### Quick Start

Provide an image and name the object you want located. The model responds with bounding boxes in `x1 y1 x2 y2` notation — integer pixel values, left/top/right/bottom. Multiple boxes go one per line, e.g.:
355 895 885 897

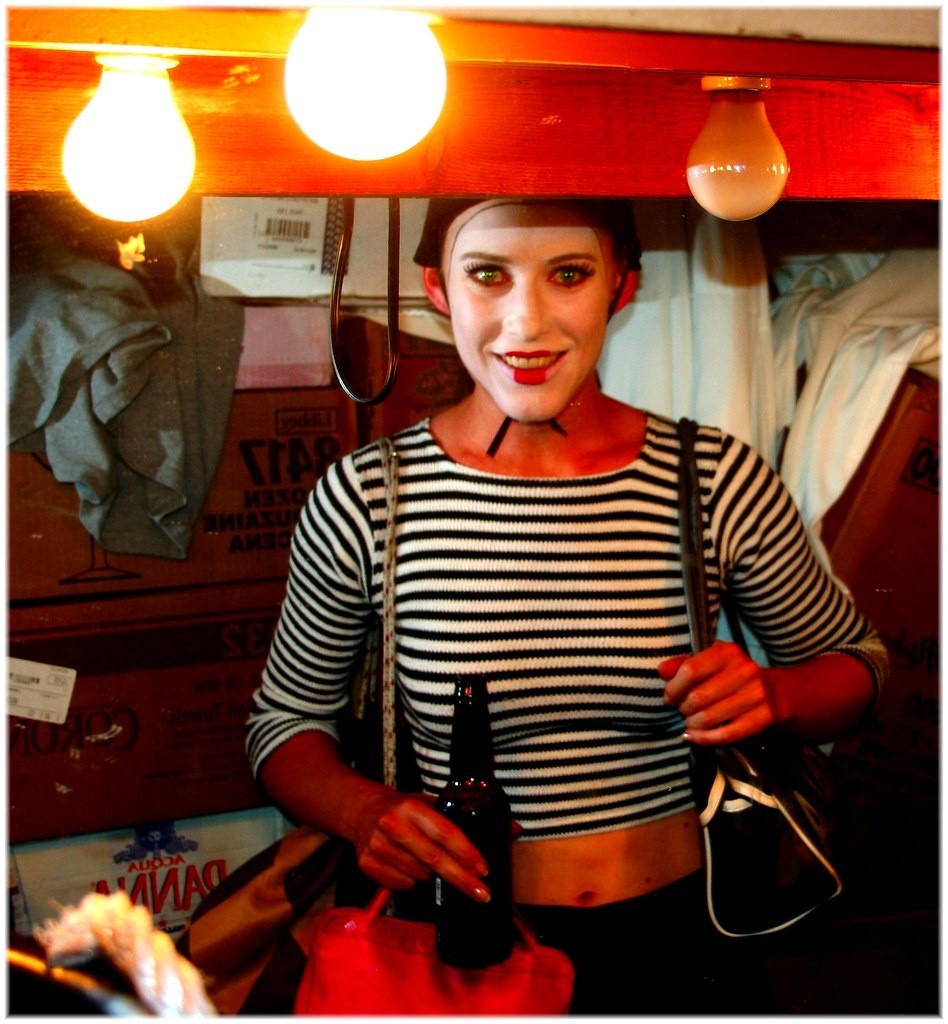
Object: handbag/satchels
678 415 846 938
177 824 393 1014
296 887 574 1017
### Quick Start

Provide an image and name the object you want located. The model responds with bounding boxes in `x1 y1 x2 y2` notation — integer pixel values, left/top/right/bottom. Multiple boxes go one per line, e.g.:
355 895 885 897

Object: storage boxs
8 191 477 969
779 362 940 810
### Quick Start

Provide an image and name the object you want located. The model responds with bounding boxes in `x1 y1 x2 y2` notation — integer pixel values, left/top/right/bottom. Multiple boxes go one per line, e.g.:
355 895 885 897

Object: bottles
435 678 516 971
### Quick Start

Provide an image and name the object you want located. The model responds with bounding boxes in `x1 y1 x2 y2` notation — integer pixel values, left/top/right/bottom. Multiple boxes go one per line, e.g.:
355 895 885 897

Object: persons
244 198 889 1017
